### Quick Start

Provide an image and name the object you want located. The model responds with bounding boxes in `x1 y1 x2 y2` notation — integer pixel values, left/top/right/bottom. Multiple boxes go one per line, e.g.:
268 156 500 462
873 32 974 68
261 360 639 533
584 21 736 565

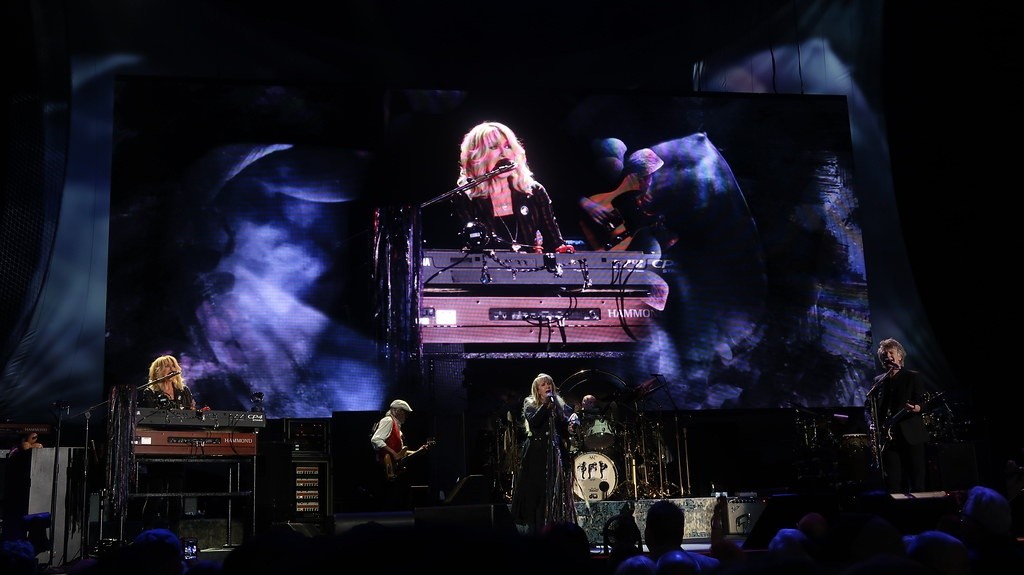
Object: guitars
867 399 916 453
384 439 437 480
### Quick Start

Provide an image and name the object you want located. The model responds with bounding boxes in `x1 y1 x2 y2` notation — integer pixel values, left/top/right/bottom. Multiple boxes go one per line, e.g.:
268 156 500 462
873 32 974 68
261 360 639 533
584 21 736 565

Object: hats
390 399 413 413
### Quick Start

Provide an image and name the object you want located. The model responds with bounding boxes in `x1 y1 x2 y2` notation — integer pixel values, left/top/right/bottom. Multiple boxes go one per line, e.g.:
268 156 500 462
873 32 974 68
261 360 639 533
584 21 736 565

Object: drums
579 415 616 449
571 452 620 502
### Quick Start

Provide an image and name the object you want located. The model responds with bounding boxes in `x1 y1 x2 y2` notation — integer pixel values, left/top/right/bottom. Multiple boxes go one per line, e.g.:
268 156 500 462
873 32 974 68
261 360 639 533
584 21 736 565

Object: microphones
169 371 181 375
547 393 554 403
885 358 899 368
495 157 518 171
651 373 663 376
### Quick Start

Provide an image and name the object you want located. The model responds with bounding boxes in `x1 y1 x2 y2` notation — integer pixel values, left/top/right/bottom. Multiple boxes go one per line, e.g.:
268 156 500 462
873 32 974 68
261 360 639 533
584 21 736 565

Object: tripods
616 396 665 501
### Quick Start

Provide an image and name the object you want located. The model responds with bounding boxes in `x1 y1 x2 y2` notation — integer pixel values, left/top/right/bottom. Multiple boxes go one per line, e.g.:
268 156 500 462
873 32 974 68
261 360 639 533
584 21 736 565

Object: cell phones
182 537 198 565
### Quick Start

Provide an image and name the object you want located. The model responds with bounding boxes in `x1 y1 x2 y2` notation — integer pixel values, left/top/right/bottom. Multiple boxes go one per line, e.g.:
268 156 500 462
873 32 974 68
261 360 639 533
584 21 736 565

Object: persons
370 399 433 512
568 394 598 426
415 120 568 255
865 338 933 474
141 354 196 408
1 468 1024 575
572 156 653 250
512 372 582 534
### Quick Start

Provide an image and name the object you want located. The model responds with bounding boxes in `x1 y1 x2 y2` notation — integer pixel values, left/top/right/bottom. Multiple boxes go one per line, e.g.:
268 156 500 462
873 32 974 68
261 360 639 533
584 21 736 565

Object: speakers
443 475 491 506
876 492 950 537
414 503 520 537
741 494 854 551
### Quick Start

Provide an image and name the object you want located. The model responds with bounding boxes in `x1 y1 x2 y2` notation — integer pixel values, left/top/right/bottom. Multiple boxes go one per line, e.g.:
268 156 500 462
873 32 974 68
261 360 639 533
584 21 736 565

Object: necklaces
494 207 521 252
